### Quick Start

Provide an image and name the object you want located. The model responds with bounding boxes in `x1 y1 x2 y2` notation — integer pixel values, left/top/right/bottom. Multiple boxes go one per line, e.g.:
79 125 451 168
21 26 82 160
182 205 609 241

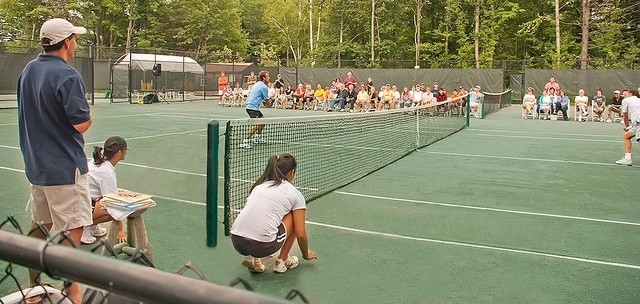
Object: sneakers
90 224 107 236
79 226 97 244
241 255 265 273
615 158 632 166
605 119 612 123
254 134 266 144
239 138 252 149
621 119 624 124
273 253 300 273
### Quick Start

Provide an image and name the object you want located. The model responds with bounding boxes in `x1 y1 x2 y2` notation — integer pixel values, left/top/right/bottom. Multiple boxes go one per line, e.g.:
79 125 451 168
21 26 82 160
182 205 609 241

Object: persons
436 88 448 117
409 85 416 98
557 90 570 121
273 86 287 109
356 86 369 112
303 83 308 93
241 70 276 148
575 89 589 122
391 85 400 114
356 81 365 97
329 80 338 99
326 85 348 112
273 73 284 109
432 81 440 97
420 83 427 93
230 153 318 273
368 87 380 111
377 84 395 114
312 83 324 110
605 89 626 123
378 86 386 101
217 71 229 105
538 88 553 120
292 83 305 110
80 134 128 246
448 89 461 117
464 85 482 118
365 78 373 96
222 83 233 108
339 84 357 113
280 83 295 110
297 84 314 111
343 72 357 90
233 82 247 108
548 88 558 114
322 87 335 111
15 18 97 304
399 87 412 115
616 90 640 166
458 85 469 118
592 88 606 122
335 77 342 92
545 76 560 95
522 87 536 120
419 86 434 116
261 82 278 109
410 83 423 116
246 70 257 95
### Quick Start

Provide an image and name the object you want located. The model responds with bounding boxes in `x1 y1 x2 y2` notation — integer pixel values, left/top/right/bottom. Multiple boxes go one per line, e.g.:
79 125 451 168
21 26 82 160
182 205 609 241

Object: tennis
414 66 420 70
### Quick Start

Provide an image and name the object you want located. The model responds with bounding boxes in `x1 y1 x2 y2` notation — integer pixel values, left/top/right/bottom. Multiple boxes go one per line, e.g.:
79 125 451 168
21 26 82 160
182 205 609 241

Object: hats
614 90 620 94
39 18 87 46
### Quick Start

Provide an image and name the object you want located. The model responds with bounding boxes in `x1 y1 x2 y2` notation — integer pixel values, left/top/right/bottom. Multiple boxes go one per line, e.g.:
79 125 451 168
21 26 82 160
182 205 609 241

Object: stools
614 112 623 123
100 195 155 262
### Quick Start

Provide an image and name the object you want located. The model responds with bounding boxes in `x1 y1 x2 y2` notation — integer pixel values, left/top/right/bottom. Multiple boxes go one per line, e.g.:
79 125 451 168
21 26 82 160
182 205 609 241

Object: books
99 190 156 211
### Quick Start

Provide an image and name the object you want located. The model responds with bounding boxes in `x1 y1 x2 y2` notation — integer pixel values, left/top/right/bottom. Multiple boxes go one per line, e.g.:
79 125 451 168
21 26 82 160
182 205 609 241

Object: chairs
222 90 482 118
592 100 606 122
522 99 538 119
553 98 570 119
575 98 588 121
539 98 551 120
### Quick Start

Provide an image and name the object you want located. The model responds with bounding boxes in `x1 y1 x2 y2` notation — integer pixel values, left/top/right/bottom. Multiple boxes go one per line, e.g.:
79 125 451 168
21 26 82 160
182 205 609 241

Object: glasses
123 148 129 151
